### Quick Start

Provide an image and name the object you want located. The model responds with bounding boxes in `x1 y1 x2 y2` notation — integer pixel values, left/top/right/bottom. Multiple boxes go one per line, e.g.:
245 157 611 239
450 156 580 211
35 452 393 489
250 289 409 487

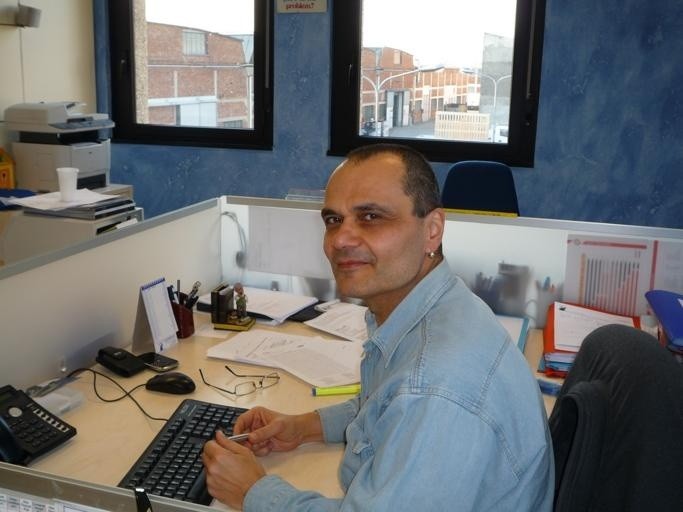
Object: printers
5 98 117 192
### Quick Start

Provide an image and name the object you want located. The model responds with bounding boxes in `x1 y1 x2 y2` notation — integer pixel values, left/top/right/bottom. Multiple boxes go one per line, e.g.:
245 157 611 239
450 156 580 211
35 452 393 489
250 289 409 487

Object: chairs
436 160 520 220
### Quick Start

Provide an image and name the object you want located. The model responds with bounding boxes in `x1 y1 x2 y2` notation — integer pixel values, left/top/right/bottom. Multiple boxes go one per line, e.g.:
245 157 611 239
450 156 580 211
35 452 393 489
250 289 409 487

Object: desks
0 195 681 511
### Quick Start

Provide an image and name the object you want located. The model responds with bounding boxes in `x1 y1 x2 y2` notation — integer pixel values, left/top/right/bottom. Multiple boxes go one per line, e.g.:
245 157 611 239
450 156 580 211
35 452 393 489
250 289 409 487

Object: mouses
146 372 196 393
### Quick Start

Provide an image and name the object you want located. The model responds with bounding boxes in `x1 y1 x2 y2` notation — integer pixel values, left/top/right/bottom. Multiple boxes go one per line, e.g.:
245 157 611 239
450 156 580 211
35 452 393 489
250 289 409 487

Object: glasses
199 365 280 397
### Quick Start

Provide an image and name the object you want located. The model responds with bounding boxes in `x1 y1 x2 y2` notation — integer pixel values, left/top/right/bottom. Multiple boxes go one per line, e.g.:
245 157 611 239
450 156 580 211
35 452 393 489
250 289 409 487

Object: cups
55 167 79 202
523 290 560 330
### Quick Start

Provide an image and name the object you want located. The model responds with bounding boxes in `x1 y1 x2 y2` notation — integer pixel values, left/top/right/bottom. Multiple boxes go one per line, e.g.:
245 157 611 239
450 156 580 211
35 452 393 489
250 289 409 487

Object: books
9 187 136 221
537 299 660 379
494 311 529 355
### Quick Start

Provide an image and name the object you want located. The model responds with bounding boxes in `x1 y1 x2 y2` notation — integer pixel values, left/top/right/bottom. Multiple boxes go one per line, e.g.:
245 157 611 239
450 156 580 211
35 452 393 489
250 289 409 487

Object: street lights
462 68 512 143
363 64 444 136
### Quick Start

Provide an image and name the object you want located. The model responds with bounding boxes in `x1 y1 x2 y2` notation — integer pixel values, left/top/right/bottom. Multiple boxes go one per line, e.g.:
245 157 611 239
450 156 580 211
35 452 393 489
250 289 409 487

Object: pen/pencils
167 280 201 309
312 383 361 396
228 433 248 443
535 277 555 292
475 272 502 297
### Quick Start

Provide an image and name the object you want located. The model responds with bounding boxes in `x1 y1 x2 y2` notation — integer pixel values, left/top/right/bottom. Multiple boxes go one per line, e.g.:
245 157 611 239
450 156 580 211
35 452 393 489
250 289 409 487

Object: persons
200 144 558 512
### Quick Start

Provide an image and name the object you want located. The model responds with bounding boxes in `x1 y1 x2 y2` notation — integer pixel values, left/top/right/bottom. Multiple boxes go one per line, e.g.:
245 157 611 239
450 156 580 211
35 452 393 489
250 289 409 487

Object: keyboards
117 399 252 505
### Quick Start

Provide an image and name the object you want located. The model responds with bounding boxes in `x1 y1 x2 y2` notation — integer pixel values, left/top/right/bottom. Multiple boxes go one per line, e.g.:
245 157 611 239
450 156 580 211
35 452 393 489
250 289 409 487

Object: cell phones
136 351 178 372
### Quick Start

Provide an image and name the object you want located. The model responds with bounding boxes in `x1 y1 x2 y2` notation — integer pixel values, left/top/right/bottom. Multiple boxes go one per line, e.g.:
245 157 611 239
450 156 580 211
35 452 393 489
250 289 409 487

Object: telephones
0 384 77 467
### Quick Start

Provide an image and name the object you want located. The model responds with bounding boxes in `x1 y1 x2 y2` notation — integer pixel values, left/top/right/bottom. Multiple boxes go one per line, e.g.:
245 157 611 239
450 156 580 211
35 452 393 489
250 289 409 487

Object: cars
488 125 508 143
